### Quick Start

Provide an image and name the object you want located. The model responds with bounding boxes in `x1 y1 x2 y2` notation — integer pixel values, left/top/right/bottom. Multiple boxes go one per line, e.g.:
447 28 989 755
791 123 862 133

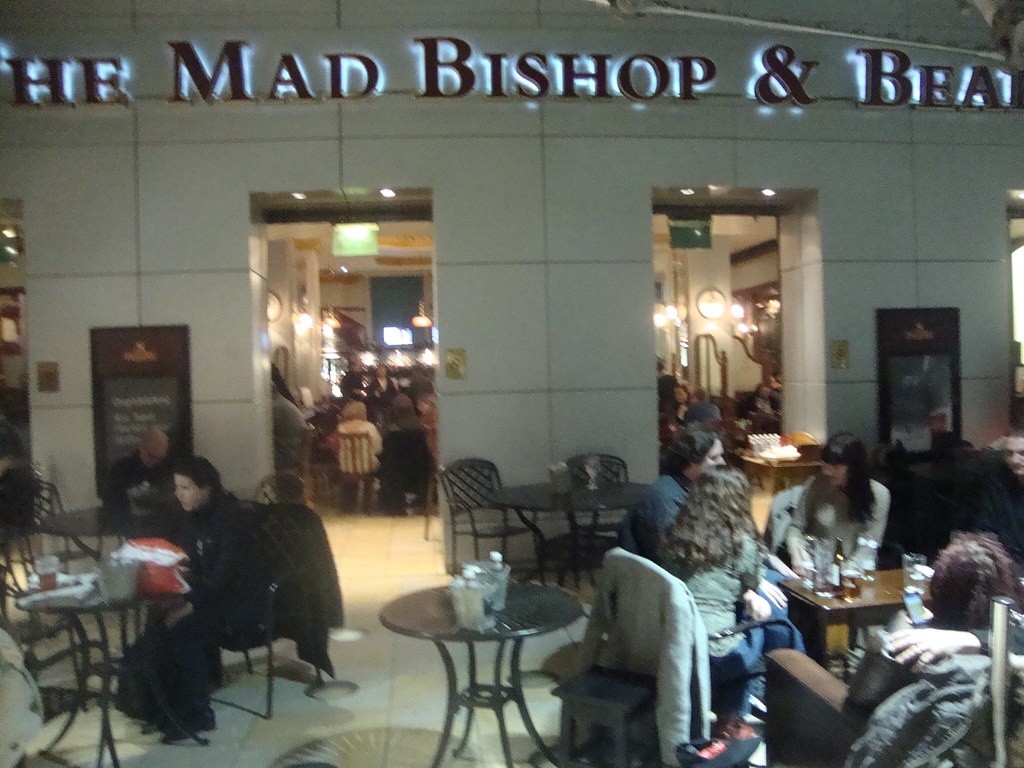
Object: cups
548 465 569 493
34 555 59 591
841 570 861 603
94 557 138 605
902 554 928 594
863 562 876 581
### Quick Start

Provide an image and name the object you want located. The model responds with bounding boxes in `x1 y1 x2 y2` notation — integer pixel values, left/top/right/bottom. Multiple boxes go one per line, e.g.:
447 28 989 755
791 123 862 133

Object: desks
740 455 821 542
14 564 209 768
379 583 582 768
776 568 933 671
489 480 654 589
37 509 164 560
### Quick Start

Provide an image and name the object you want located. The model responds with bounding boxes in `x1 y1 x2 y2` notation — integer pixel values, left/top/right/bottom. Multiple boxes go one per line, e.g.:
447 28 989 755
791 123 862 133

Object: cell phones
902 592 929 629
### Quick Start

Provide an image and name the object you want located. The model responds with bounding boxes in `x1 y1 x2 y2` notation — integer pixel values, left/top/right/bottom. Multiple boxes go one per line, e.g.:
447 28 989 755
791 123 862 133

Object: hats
391 394 420 431
684 402 722 422
142 425 168 459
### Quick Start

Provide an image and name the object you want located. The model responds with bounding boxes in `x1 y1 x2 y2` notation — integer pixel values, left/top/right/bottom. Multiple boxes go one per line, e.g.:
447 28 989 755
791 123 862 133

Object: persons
269 355 436 515
624 361 1024 767
0 412 272 743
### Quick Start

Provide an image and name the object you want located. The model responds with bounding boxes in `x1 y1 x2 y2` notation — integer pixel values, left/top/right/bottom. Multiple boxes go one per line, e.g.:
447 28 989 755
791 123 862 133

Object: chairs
440 459 543 576
552 427 1024 768
335 432 381 510
0 460 320 768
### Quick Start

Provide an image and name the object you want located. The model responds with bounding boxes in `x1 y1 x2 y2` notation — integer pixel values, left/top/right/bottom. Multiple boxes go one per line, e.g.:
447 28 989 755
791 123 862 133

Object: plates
759 452 801 461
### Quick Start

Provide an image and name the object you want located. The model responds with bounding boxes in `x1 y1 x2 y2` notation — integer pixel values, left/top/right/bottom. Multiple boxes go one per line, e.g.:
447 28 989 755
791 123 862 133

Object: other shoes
157 704 216 743
717 719 752 740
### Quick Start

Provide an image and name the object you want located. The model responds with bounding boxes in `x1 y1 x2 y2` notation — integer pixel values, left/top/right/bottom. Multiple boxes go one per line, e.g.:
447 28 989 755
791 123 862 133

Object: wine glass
584 456 601 490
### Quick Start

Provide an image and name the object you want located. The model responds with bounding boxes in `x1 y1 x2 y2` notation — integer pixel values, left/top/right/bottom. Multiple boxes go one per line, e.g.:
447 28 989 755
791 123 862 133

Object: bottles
748 433 780 457
829 538 847 595
484 552 507 610
454 571 484 629
